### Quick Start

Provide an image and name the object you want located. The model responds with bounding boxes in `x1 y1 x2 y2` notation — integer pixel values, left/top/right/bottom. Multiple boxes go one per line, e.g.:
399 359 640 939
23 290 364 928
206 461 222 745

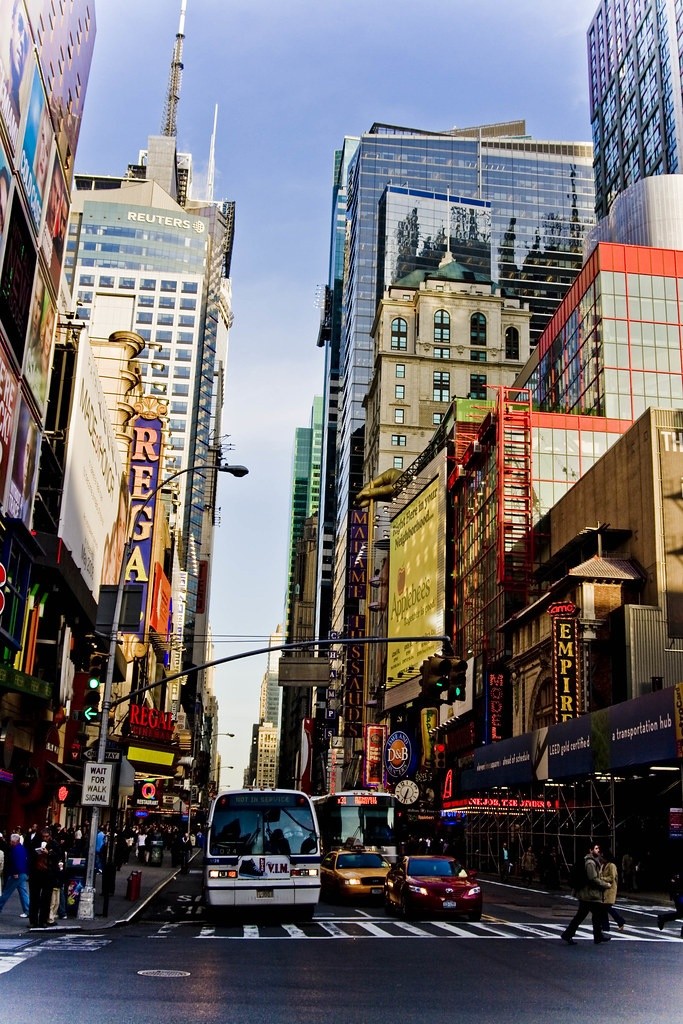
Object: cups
421 707 438 768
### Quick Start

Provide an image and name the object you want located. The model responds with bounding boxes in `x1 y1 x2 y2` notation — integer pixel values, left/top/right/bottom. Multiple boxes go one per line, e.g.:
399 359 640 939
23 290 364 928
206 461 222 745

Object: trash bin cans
66 865 96 918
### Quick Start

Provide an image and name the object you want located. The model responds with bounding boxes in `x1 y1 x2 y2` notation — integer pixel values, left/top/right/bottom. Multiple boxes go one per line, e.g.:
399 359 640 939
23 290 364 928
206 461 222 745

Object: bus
310 790 397 867
206 786 321 922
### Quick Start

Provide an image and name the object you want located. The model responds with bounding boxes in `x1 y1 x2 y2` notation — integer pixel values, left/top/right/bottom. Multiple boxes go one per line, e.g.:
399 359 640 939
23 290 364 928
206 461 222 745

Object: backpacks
571 857 599 889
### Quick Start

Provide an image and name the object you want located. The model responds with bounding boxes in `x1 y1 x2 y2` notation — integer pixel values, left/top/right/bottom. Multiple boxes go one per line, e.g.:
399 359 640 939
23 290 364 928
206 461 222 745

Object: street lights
77 463 249 920
188 732 234 832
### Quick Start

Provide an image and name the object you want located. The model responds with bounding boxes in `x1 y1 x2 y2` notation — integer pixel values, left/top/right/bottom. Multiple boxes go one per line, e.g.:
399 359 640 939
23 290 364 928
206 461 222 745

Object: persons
405 833 683 944
0 820 204 928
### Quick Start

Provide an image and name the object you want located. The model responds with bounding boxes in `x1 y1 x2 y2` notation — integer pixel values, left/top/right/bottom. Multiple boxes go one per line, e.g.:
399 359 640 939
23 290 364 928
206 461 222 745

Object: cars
320 837 394 904
385 856 484 923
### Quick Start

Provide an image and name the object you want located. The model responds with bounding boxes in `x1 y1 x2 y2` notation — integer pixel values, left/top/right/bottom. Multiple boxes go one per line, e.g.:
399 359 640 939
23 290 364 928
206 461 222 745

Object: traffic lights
87 654 103 690
418 656 468 706
56 782 82 809
434 744 445 769
82 689 101 723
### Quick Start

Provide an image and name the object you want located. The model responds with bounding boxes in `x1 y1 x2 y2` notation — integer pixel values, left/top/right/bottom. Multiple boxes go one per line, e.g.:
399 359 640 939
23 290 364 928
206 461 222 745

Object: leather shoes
594 936 611 944
561 933 578 945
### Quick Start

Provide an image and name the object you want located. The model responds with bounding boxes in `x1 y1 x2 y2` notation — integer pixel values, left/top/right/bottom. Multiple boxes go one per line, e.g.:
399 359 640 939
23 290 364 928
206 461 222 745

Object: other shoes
99 892 114 897
27 923 47 928
62 916 68 919
618 921 624 930
116 867 120 871
680 926 683 938
658 914 665 930
47 922 58 926
19 913 29 918
